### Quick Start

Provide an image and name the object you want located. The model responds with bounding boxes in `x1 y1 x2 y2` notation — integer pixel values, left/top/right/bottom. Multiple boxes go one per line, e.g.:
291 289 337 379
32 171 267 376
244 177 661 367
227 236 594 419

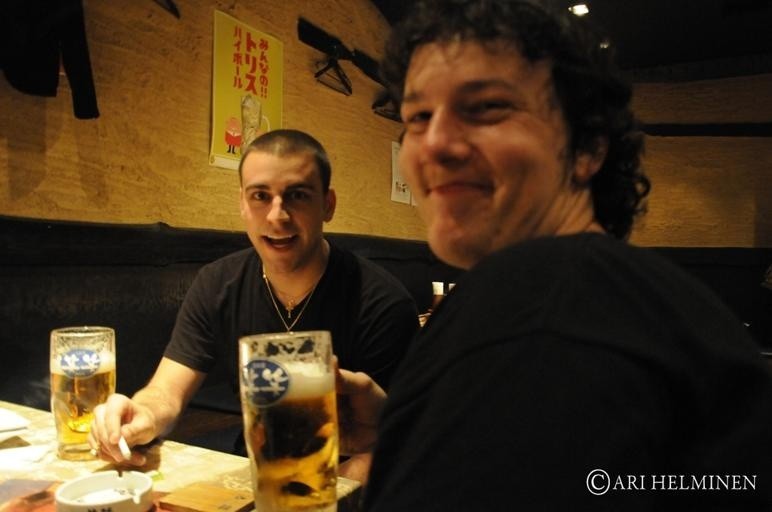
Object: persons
89 129 421 504
333 0 772 512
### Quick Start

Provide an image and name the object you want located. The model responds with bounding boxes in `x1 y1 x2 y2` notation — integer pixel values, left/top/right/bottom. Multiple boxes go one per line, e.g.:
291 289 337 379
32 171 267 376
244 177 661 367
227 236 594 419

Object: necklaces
263 263 326 332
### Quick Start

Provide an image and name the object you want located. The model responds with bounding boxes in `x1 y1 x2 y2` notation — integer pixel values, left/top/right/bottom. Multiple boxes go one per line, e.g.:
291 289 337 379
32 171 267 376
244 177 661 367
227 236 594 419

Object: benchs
1 210 771 469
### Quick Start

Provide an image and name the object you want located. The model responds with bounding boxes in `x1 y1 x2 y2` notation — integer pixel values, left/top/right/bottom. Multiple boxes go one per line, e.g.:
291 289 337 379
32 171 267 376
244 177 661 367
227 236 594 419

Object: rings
90 440 102 457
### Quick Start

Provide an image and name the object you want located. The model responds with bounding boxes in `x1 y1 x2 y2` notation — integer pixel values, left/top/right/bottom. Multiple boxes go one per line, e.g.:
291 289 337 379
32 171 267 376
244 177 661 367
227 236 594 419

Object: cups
239 330 339 512
50 325 119 460
432 294 445 310
